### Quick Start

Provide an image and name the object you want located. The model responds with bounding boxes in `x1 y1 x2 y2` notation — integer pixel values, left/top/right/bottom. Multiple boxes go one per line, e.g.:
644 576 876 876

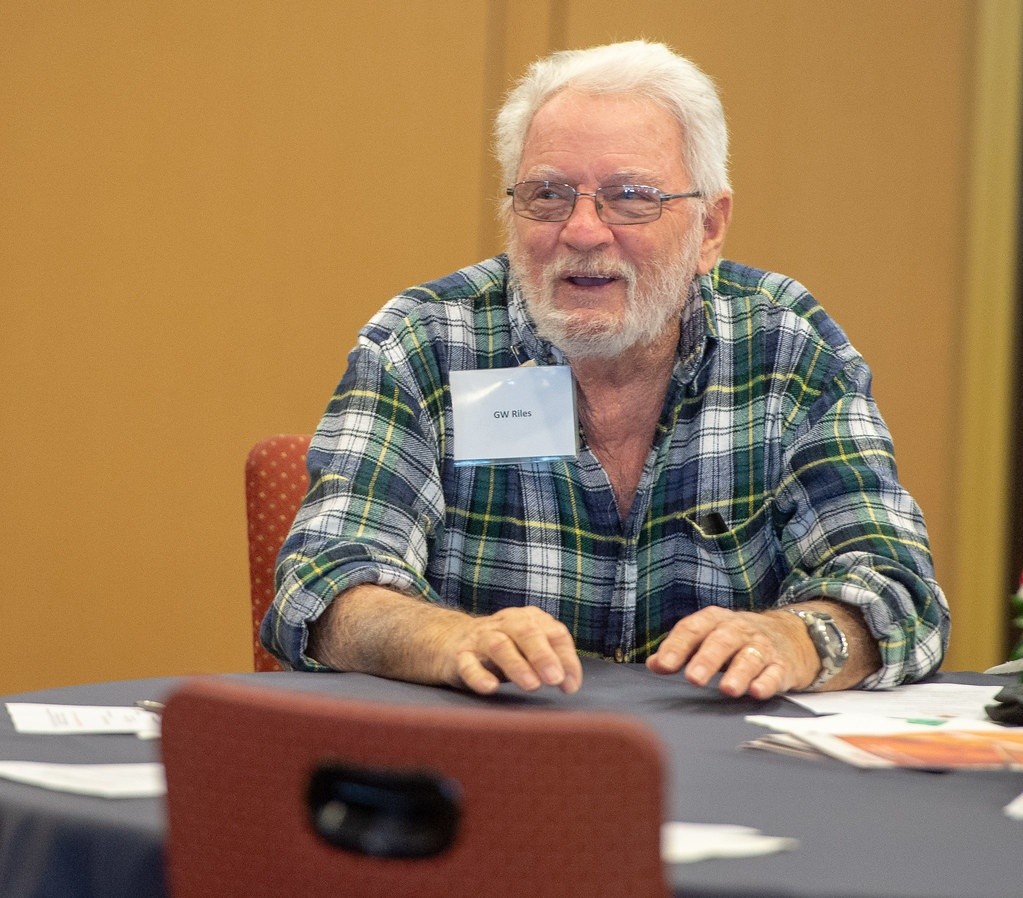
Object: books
739 683 1023 770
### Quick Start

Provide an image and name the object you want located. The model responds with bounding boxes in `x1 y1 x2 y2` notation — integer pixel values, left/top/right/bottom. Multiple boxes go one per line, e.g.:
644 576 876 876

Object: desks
0 662 1023 897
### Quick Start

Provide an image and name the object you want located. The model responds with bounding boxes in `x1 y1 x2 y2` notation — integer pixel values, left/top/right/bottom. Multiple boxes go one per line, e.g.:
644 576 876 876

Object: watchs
779 607 848 693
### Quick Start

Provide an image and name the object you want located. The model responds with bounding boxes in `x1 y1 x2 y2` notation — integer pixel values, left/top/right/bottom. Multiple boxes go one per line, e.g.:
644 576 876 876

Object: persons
258 37 952 699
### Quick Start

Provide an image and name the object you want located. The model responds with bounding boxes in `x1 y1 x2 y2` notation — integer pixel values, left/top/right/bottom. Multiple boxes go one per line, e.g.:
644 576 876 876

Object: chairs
159 686 668 898
244 437 312 676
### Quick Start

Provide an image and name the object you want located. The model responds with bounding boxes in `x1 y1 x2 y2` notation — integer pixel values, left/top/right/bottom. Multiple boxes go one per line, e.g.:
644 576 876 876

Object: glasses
506 181 705 226
